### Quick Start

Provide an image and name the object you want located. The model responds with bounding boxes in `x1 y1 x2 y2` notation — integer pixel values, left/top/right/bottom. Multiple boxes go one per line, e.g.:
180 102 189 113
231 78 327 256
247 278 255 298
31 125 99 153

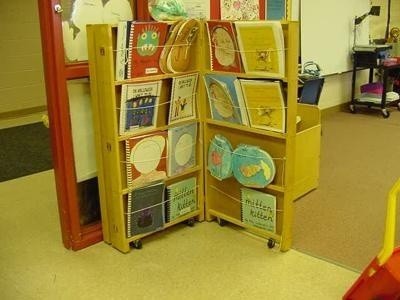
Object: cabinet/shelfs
86 20 300 253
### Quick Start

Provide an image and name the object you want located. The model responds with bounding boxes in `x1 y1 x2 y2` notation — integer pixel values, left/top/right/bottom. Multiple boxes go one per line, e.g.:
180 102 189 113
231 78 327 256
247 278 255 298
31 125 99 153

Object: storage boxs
294 104 321 201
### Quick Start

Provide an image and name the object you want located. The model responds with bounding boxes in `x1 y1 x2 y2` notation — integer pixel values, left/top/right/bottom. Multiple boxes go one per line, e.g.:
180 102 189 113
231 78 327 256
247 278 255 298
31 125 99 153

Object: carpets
0 121 54 182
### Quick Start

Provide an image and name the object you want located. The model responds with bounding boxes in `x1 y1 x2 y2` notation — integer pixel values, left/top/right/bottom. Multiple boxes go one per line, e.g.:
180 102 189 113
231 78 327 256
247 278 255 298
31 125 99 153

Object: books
158 18 201 74
202 74 250 127
116 21 169 80
119 80 162 136
125 131 168 188
165 176 198 223
168 73 198 125
235 21 286 77
206 133 233 182
231 144 276 189
238 79 287 133
238 187 277 234
206 21 242 74
126 181 169 237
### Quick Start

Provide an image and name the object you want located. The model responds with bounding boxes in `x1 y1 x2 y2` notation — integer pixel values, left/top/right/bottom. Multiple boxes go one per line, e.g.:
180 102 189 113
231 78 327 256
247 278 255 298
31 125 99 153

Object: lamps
352 6 380 50
